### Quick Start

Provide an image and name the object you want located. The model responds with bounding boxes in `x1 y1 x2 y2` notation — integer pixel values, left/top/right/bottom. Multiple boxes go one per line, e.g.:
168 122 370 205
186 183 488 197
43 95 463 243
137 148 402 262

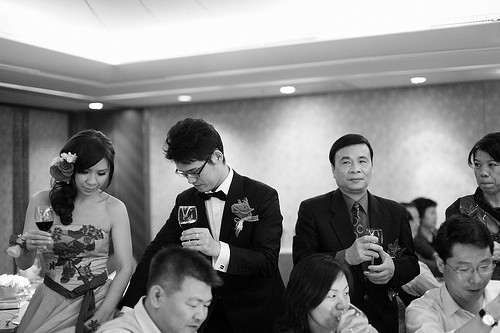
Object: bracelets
7 234 31 257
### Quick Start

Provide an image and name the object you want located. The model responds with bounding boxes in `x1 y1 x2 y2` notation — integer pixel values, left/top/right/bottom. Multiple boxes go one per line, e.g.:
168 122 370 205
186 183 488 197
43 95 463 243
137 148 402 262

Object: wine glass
178 205 197 242
34 206 56 253
362 228 383 274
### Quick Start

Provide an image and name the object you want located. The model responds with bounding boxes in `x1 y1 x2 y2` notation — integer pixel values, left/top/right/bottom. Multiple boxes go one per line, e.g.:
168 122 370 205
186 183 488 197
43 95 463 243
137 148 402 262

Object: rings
196 233 198 239
196 240 198 245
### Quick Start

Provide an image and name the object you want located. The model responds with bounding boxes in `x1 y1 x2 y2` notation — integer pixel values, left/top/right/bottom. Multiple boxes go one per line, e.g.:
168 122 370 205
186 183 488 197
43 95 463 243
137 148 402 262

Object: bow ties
202 191 227 201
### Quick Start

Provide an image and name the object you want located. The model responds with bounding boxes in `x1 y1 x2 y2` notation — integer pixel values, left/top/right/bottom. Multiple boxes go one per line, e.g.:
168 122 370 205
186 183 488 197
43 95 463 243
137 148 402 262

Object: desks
0 277 46 333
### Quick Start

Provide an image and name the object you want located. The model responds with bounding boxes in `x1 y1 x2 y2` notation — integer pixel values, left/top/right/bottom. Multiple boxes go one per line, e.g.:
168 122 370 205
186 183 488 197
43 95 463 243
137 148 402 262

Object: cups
336 302 368 332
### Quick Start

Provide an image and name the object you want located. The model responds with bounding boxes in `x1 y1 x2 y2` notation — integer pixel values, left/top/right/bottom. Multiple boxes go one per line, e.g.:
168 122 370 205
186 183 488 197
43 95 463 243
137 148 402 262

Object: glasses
175 148 219 179
444 259 496 275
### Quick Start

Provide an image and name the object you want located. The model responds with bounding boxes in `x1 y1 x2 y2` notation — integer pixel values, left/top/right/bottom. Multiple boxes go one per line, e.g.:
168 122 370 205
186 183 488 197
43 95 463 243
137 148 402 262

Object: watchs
479 309 498 328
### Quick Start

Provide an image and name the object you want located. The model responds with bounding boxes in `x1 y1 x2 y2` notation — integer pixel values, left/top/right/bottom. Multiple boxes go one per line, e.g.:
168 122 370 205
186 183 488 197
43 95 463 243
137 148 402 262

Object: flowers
48 152 77 184
231 198 259 236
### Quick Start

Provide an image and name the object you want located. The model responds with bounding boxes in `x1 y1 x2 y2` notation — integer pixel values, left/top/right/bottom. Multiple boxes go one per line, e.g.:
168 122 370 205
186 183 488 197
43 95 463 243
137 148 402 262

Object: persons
16 129 133 333
273 253 378 333
95 244 223 333
446 132 500 281
292 134 420 333
114 117 285 333
405 212 500 333
398 203 444 308
410 197 439 250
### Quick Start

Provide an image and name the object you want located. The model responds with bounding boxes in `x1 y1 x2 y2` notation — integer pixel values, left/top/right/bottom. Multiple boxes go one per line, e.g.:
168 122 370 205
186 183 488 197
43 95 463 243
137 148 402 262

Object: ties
351 201 365 240
